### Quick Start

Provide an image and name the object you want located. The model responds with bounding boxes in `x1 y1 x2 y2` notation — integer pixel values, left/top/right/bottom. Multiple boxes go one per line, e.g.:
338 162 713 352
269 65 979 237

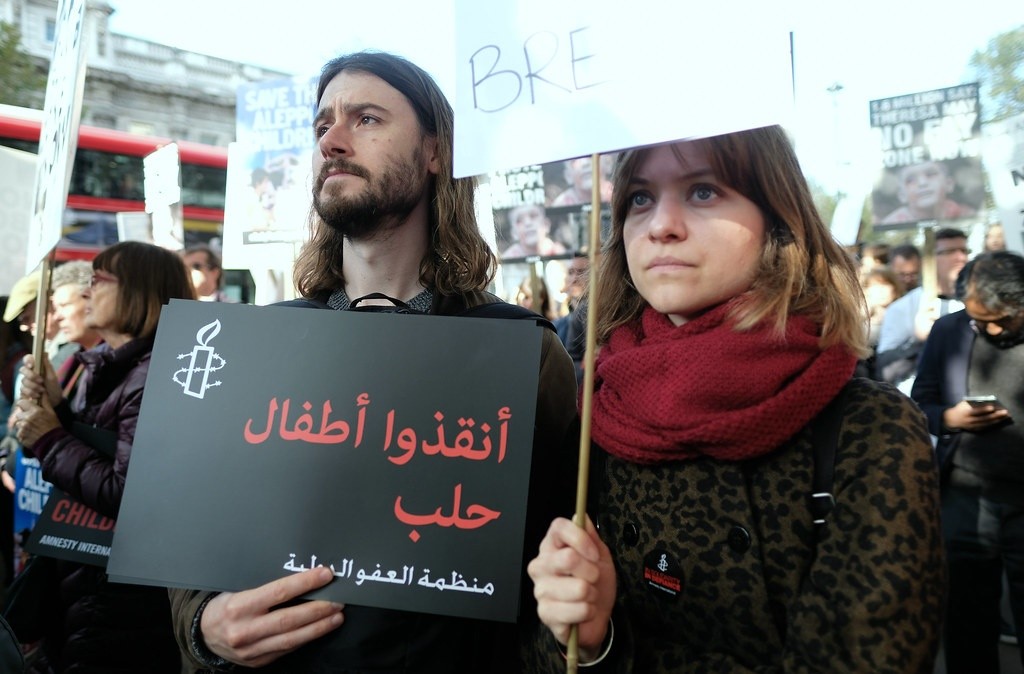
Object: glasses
187 262 214 270
936 247 971 257
969 309 1020 333
89 275 120 289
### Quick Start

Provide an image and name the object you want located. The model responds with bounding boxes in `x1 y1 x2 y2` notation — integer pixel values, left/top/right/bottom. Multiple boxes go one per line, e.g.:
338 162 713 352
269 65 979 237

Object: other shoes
999 622 1017 643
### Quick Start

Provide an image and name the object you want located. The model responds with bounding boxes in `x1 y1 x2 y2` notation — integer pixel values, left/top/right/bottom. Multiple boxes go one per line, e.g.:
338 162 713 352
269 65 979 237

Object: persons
0 240 231 674
520 256 586 343
503 155 615 257
847 222 1023 674
167 51 577 674
243 168 276 227
886 160 971 223
525 127 946 674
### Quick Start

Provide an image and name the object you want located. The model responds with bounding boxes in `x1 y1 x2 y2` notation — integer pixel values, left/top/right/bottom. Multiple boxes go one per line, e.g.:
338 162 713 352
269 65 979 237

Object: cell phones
966 395 1011 418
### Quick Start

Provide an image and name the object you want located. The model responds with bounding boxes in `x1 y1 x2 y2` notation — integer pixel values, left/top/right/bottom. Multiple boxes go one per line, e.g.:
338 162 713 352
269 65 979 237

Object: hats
3 273 38 322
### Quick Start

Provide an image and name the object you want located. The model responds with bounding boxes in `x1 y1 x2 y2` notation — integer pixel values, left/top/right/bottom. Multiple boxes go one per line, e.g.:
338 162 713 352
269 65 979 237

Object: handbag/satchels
0 614 26 674
